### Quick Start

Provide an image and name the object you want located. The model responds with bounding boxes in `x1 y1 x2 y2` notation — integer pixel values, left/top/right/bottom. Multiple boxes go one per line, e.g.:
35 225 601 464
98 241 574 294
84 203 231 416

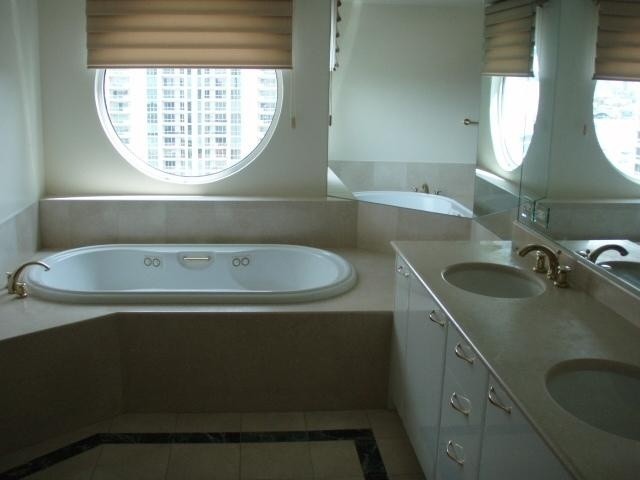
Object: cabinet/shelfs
477 369 569 479
388 250 409 453
431 317 488 478
405 270 447 475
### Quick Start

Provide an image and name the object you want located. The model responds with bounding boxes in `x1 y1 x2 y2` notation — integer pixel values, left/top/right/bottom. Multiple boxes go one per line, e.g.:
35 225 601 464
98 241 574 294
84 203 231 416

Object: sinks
595 260 639 292
545 357 640 442
441 261 547 299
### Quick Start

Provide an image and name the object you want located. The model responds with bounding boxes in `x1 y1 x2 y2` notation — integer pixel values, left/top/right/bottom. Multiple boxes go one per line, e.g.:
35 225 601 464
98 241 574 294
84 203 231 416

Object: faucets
421 182 429 194
7 260 50 297
517 244 560 280
587 244 631 265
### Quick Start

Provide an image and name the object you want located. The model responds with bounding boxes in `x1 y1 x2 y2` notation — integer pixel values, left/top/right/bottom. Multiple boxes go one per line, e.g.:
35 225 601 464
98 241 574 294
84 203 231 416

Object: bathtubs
352 190 476 219
21 242 360 305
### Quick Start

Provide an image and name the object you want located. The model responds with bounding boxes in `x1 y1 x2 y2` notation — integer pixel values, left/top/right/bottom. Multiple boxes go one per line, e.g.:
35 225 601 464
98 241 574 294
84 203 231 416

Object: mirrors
327 1 640 298
